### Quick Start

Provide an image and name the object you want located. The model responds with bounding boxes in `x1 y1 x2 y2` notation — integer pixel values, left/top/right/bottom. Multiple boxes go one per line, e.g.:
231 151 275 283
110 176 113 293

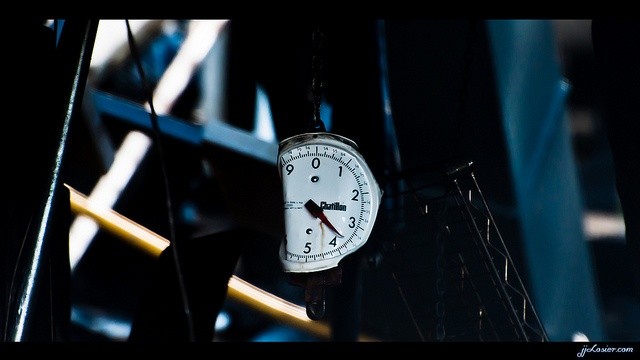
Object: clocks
279 131 382 322
